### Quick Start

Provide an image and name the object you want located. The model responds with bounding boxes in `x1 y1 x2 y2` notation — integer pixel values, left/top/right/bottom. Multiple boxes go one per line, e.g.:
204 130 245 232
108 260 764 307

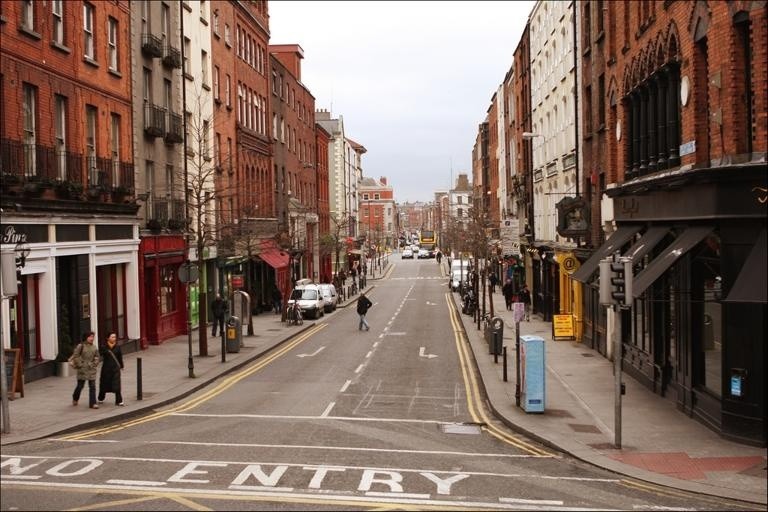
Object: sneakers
99 401 103 404
118 403 123 406
73 399 78 405
92 404 99 408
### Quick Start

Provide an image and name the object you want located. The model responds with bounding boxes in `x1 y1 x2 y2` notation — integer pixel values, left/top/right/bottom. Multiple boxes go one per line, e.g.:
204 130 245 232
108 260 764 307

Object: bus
418 229 437 250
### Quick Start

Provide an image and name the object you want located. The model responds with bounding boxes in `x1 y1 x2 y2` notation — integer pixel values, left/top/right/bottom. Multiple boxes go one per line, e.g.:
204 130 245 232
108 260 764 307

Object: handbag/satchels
69 355 79 368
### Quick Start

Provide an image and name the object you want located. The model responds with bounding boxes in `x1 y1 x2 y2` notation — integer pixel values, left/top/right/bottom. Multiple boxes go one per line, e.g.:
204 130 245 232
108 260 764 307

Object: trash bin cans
489 317 503 354
226 316 241 353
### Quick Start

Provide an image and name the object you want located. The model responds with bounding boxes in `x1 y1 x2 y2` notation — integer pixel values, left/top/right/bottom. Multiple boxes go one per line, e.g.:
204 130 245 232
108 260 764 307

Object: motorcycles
459 285 476 316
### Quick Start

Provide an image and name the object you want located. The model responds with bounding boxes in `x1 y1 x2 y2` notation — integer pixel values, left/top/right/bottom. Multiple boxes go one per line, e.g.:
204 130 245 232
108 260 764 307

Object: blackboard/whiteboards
4 348 19 395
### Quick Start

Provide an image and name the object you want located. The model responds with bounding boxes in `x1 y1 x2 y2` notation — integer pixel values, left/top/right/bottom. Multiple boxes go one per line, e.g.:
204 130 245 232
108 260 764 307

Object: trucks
446 259 470 288
317 282 340 313
285 277 326 321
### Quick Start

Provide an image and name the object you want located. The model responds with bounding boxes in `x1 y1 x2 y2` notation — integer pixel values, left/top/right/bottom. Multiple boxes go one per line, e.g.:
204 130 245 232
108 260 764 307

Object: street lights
367 197 377 258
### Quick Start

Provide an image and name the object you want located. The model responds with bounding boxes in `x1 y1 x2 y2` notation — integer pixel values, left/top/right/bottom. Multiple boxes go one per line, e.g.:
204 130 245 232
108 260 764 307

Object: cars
398 235 435 260
449 269 471 292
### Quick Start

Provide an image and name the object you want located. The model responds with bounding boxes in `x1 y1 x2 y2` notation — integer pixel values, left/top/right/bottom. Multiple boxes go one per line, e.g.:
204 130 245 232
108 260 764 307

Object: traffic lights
608 258 633 308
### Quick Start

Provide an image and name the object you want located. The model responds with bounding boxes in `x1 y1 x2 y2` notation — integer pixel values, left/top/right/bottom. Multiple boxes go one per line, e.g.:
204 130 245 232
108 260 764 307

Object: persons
96 331 125 406
209 293 226 338
357 292 371 329
435 251 441 262
460 265 531 323
271 259 366 314
72 331 99 408
